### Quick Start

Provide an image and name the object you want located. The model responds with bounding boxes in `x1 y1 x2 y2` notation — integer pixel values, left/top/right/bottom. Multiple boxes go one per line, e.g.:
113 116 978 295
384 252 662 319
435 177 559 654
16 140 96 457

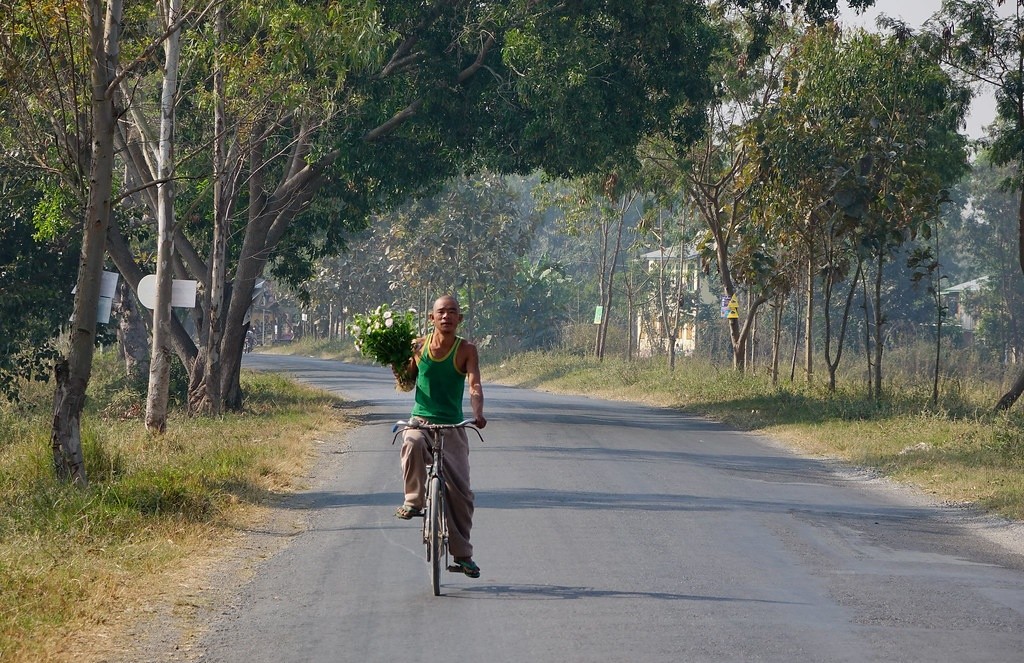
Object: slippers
458 559 480 579
395 505 419 520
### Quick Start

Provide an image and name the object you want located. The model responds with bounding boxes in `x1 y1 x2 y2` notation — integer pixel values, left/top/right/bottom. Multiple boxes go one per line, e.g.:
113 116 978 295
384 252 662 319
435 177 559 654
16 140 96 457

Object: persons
392 295 487 578
247 325 253 350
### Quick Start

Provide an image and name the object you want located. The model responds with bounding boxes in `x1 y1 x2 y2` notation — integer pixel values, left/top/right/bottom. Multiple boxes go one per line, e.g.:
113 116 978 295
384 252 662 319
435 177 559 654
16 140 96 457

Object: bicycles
244 332 259 355
391 417 485 597
